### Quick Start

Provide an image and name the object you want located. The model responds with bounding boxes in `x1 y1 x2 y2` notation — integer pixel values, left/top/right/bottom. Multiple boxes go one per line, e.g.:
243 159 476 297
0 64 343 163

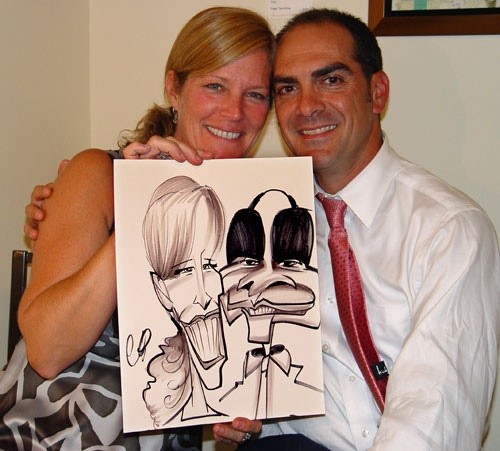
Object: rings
242 432 251 443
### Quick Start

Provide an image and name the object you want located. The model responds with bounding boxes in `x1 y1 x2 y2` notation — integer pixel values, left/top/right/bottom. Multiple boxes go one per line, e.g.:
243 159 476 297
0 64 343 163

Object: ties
315 192 390 415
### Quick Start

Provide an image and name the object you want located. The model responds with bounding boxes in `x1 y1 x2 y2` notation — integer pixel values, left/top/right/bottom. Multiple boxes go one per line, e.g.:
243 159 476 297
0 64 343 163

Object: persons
19 5 499 451
0 4 278 451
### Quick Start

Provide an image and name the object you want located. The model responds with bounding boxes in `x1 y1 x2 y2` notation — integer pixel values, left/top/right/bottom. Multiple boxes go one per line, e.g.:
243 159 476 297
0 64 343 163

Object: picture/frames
365 0 500 37
